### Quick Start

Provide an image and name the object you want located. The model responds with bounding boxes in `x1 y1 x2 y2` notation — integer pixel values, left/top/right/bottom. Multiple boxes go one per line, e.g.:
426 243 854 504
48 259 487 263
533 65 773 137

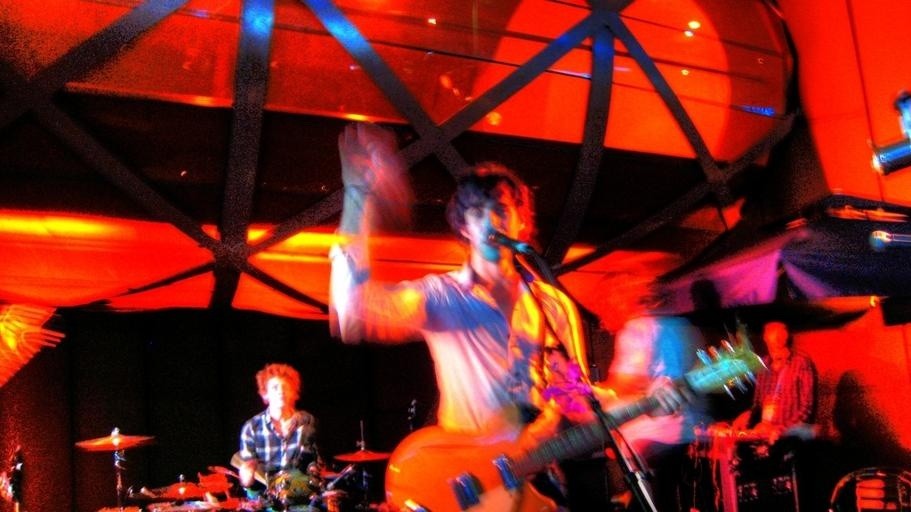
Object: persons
329 122 592 512
748 321 819 457
238 365 322 512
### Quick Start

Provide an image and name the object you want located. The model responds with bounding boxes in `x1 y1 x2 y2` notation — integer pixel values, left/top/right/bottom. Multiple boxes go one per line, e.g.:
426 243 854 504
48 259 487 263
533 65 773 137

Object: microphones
486 230 531 254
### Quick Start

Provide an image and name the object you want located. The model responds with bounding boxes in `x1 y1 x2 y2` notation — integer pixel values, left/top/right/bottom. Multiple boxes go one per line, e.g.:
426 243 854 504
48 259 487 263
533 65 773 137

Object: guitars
381 333 772 511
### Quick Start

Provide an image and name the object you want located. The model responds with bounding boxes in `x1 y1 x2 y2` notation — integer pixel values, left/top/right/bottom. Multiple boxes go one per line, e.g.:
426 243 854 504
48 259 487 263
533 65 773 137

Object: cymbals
332 447 391 464
141 480 215 500
70 433 157 453
